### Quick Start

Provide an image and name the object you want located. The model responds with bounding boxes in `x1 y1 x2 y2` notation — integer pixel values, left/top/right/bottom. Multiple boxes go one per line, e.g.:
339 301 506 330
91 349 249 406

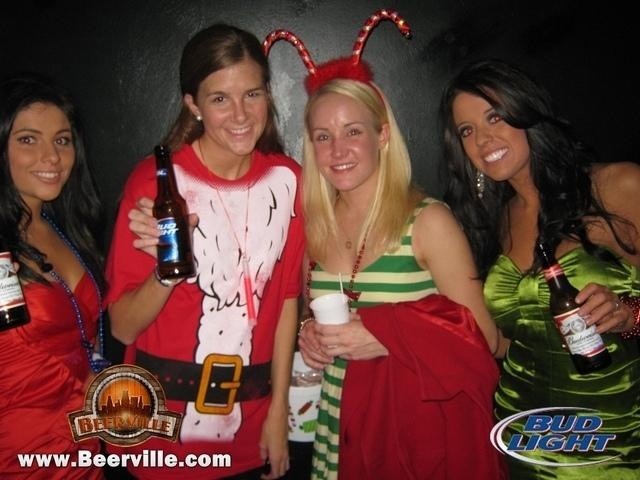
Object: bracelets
296 315 316 339
153 264 179 289
618 295 640 341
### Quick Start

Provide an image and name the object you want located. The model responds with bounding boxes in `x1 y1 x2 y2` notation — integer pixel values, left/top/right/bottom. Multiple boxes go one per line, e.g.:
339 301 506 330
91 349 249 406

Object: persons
1 90 114 480
296 73 503 479
435 57 640 480
100 20 307 480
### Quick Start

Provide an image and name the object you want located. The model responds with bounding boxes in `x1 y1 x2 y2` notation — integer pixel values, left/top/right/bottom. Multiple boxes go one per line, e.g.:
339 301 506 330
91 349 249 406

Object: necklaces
199 134 257 328
19 207 106 376
344 233 355 252
306 237 367 314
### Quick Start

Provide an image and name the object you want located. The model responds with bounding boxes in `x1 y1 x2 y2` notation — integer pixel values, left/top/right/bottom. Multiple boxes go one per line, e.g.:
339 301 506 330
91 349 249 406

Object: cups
308 294 351 324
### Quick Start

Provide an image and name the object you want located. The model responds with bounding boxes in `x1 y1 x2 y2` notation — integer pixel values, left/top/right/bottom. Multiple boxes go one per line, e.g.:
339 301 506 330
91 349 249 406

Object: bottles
152 142 193 279
534 241 612 375
1 242 30 331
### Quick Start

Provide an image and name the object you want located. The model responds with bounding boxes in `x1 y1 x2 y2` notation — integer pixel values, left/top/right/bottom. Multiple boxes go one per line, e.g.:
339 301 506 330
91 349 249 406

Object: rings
614 300 620 310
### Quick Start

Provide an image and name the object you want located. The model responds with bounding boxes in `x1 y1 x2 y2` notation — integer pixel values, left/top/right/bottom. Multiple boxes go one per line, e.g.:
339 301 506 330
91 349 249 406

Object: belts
133 347 271 414
